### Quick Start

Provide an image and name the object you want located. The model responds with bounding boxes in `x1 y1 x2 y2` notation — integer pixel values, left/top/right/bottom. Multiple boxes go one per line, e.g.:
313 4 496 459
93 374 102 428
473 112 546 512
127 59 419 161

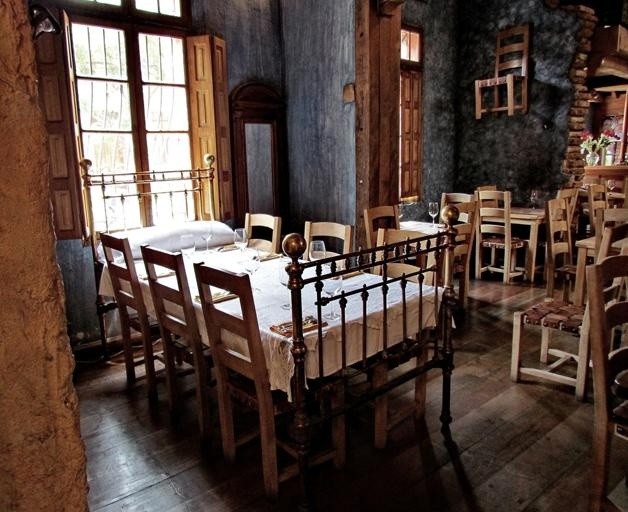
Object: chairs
471 25 534 122
469 183 520 284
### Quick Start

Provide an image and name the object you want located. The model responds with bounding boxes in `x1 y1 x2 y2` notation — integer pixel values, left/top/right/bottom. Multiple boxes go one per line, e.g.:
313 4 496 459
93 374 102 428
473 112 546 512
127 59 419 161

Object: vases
585 152 601 167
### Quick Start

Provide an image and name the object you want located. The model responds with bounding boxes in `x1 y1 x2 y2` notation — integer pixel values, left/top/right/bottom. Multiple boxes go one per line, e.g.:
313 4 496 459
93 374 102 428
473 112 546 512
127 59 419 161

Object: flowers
577 127 621 155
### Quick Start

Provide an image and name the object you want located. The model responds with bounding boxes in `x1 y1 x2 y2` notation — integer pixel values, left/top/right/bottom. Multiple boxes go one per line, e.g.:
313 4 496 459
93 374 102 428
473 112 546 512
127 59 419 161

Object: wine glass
167 229 214 267
324 274 343 320
429 202 439 228
229 225 291 311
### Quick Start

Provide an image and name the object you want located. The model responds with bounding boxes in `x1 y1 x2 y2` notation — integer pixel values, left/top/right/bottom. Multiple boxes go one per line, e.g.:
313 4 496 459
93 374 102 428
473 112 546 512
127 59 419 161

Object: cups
607 180 615 190
309 240 326 262
